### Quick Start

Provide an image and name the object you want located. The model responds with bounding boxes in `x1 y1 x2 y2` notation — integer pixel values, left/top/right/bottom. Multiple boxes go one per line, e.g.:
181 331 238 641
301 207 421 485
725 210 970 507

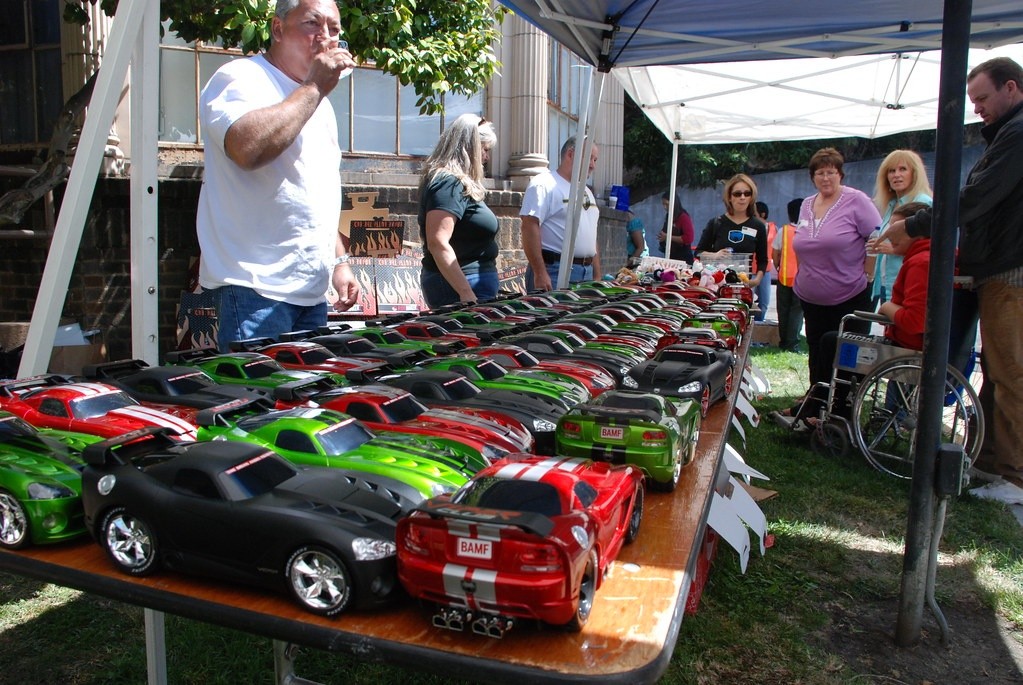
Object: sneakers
967 478 1023 506
965 461 1002 482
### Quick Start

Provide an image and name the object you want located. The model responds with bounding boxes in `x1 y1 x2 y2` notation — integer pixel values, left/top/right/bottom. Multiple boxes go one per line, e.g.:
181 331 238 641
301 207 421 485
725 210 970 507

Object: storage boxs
683 356 772 614
46 344 108 375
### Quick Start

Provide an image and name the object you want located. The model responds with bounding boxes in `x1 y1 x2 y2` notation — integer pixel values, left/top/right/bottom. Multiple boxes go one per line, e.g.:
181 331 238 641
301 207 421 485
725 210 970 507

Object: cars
0 268 750 638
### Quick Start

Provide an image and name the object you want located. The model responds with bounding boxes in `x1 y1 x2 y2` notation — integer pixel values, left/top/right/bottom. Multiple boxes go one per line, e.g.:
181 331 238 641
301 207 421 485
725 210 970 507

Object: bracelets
334 253 350 266
865 271 874 280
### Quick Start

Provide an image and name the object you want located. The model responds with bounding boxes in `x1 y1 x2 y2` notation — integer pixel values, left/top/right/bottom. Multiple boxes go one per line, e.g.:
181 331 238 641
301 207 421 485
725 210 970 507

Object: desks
0 317 755 685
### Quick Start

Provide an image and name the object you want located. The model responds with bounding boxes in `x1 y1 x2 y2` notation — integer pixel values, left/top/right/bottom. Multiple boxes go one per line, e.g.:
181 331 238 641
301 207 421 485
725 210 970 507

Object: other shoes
900 414 917 429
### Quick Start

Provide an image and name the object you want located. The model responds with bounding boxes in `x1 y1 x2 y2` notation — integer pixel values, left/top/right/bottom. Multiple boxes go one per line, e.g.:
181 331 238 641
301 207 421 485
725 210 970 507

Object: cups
324 40 352 79
502 180 514 192
608 196 618 209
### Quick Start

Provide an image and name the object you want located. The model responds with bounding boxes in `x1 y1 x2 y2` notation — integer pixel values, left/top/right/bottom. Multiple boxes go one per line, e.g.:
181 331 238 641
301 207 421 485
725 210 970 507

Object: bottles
866 226 881 257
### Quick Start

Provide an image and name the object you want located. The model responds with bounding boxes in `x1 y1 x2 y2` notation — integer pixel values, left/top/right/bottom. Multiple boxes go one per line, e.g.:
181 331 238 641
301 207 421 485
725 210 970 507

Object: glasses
731 190 751 197
478 117 486 126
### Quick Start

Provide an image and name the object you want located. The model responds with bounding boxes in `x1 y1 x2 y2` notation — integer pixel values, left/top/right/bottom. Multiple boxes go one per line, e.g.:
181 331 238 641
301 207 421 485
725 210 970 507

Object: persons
626 210 650 270
792 147 883 403
418 114 500 313
751 201 780 321
694 173 768 289
780 202 958 430
865 149 933 336
770 198 805 355
519 134 601 296
656 191 694 269
957 56 1023 505
196 0 360 353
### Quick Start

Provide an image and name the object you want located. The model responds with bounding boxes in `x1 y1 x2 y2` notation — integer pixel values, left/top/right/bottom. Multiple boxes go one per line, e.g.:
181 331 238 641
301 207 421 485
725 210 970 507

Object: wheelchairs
767 285 987 488
